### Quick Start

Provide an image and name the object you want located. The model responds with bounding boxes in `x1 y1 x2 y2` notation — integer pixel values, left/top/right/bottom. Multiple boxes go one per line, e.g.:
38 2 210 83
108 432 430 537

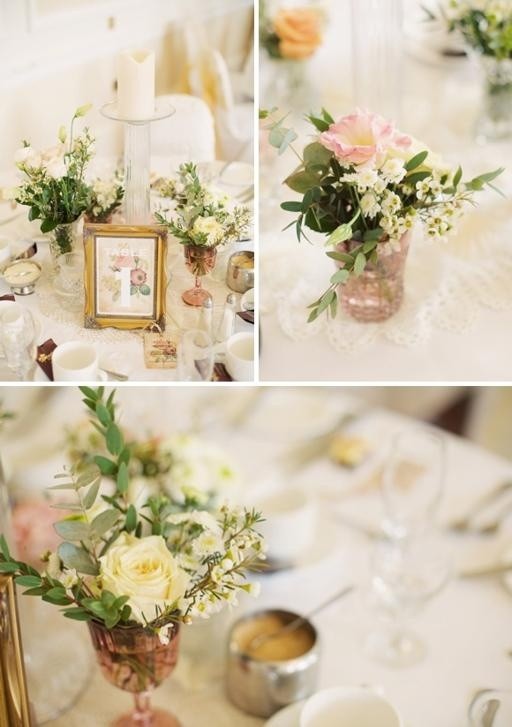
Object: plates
52 278 85 297
263 704 299 727
32 365 108 381
0 304 36 358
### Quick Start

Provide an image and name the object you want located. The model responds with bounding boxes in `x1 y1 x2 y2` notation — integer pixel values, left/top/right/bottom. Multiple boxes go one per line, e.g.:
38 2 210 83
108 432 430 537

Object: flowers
258 98 510 323
440 1 512 89
10 103 128 254
259 0 331 68
0 385 270 691
149 158 253 275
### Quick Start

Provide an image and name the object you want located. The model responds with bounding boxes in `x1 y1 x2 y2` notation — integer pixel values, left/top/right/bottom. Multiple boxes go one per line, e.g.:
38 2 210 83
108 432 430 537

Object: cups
224 609 320 715
52 343 98 382
299 689 400 726
0 300 23 341
58 252 86 290
212 332 254 382
226 251 254 293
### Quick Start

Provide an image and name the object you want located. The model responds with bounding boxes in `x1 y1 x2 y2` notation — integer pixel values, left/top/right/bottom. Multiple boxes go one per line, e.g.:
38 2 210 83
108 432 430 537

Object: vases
476 51 511 87
326 232 413 322
43 221 84 295
86 620 189 727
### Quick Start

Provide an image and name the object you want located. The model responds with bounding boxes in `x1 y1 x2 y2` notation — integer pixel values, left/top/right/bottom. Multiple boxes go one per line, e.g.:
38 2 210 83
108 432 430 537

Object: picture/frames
81 222 168 335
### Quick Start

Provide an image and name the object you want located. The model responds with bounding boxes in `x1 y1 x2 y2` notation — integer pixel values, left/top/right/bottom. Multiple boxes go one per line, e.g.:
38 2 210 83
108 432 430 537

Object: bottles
193 294 237 347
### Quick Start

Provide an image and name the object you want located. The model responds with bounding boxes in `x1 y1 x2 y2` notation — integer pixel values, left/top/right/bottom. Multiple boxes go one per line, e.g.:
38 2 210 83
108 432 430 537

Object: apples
131 269 146 286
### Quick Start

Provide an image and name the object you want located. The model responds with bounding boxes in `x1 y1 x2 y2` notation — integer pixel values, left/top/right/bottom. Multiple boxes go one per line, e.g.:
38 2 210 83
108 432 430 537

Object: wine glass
84 613 181 727
